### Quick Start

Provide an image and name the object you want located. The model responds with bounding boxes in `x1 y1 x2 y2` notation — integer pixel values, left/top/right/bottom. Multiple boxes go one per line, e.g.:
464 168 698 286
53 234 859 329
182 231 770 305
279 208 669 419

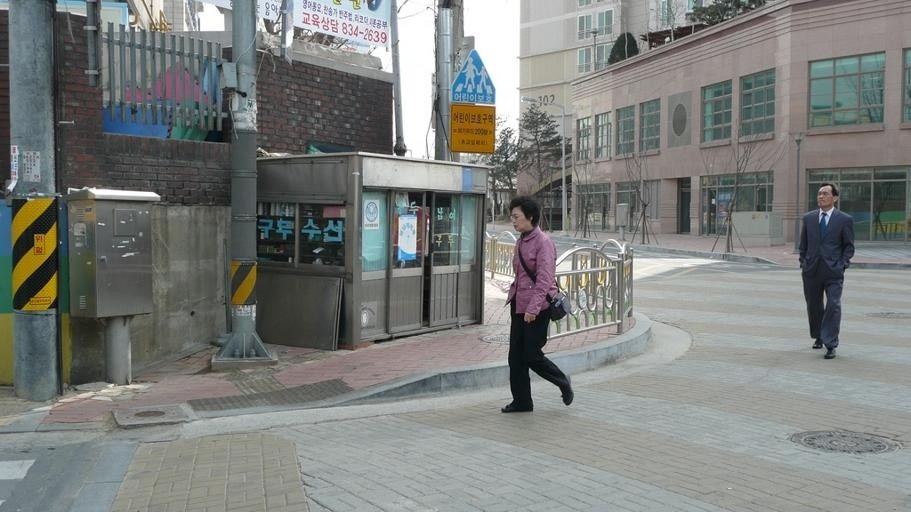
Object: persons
501 196 575 412
798 183 855 357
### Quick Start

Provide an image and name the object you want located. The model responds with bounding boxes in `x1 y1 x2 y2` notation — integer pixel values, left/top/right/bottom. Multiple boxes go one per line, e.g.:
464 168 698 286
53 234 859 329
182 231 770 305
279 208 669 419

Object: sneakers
560 373 575 406
501 396 535 413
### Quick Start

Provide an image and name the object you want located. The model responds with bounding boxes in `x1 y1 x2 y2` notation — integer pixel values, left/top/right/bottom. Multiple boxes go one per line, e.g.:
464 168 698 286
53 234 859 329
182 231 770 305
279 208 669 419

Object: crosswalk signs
449 47 496 106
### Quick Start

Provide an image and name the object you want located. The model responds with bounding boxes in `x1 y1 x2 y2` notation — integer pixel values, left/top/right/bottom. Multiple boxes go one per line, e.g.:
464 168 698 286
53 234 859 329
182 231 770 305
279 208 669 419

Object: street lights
518 93 567 235
786 127 810 255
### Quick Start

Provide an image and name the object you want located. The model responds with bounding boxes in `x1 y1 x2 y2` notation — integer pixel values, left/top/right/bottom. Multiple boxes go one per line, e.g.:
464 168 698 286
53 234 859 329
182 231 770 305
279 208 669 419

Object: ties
820 213 829 237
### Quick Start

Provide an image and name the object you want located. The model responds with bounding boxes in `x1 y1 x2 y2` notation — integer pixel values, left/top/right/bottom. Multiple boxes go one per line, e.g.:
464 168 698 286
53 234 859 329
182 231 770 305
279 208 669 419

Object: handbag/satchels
549 291 572 322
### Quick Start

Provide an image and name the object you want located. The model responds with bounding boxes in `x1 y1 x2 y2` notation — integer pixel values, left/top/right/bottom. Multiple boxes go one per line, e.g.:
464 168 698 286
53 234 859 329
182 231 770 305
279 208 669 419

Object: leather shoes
825 347 836 359
812 339 823 349
